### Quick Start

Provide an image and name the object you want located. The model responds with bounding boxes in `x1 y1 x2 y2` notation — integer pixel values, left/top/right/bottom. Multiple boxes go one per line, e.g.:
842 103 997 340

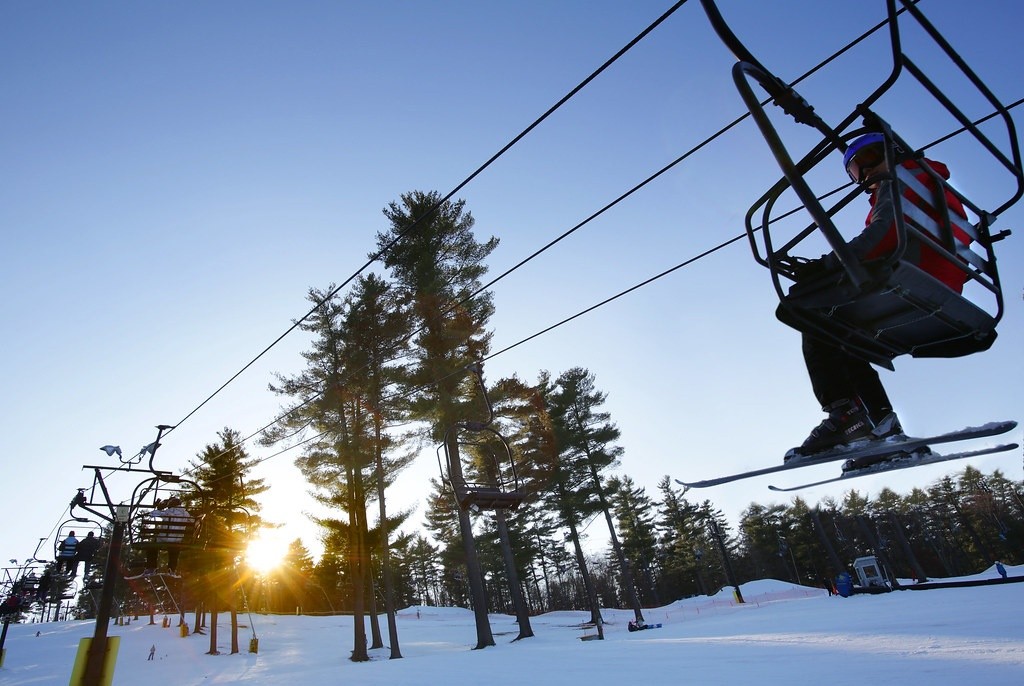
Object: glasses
847 143 885 185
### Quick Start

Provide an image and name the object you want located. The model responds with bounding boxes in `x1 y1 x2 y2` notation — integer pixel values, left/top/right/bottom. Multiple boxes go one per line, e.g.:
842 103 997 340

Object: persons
0 530 99 637
145 498 191 577
824 576 834 597
148 644 155 661
628 617 647 632
784 131 969 472
995 561 1007 578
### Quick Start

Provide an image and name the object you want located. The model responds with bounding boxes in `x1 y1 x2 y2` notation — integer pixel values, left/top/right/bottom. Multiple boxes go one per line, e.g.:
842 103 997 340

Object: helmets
843 133 904 182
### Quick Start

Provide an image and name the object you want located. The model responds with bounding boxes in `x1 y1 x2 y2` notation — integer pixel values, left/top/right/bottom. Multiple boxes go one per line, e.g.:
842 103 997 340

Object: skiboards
672 420 1018 490
125 572 181 582
768 443 1021 491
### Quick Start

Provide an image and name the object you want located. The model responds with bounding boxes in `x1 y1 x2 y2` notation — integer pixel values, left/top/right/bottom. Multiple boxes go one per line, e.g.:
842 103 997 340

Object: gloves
797 257 825 280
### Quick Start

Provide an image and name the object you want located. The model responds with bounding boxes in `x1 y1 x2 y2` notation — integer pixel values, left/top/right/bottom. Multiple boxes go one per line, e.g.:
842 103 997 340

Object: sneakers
842 413 913 471
784 401 878 461
